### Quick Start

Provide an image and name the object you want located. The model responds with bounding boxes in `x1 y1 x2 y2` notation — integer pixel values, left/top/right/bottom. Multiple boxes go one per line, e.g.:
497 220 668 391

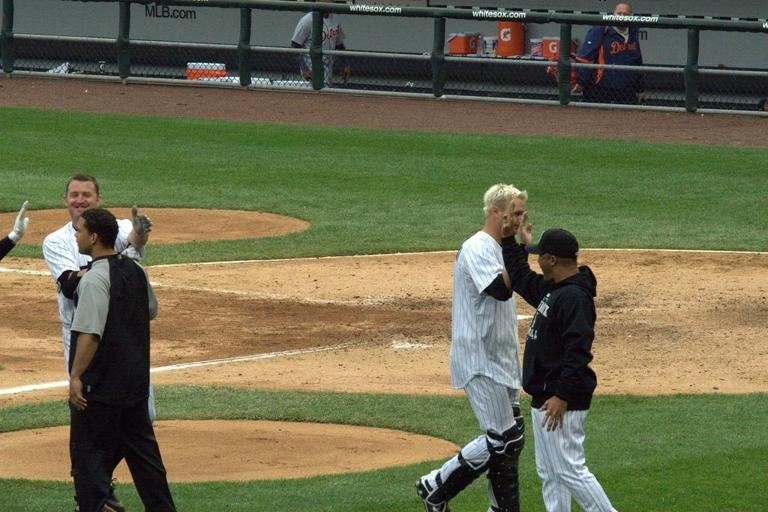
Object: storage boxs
185 60 224 79
448 31 480 54
538 34 579 62
496 21 527 56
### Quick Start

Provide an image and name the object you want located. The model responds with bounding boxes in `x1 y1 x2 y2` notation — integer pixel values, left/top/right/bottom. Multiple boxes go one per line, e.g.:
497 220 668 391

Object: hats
525 229 578 259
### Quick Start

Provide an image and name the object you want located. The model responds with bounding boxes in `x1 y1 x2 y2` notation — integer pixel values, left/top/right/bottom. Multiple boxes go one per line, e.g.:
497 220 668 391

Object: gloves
8 199 30 243
131 205 154 234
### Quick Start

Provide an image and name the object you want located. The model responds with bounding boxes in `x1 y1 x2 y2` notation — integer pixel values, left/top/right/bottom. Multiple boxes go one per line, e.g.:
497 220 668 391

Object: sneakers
415 469 450 512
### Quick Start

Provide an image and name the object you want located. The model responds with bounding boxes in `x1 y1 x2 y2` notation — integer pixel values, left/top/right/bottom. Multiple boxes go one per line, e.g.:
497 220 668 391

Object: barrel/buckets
483 36 497 53
529 37 543 58
496 20 530 58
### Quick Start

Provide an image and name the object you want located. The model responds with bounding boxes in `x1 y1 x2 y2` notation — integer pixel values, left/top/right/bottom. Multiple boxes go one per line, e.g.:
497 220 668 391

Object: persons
65 207 181 512
37 171 158 512
284 8 353 85
499 198 618 512
1 197 33 261
572 3 648 105
412 181 536 512
